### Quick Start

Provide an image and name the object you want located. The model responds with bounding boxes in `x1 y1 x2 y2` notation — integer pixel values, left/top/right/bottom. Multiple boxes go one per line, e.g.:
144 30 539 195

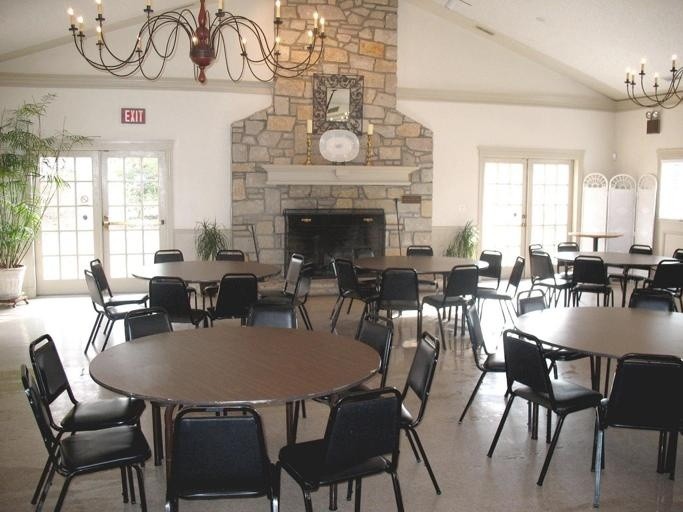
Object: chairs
571 254 614 307
590 349 681 509
257 254 305 299
163 407 277 512
243 303 309 418
472 250 501 305
526 253 572 308
202 249 244 311
643 256 683 311
83 270 147 355
330 255 353 318
274 386 408 512
513 289 597 392
458 306 533 427
122 308 173 340
607 243 652 308
623 286 670 311
88 259 148 333
374 267 419 344
148 276 208 329
527 242 546 251
207 273 258 328
486 329 602 486
301 315 395 419
556 242 581 274
421 265 478 351
330 258 376 334
345 332 443 502
347 246 380 314
672 248 682 261
153 249 197 310
21 365 147 511
291 265 314 330
405 243 437 293
474 255 525 325
26 333 146 505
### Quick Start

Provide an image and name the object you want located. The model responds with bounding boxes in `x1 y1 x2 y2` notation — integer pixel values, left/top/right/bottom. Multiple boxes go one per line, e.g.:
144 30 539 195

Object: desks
134 259 281 283
512 304 683 393
568 231 624 251
580 249 678 307
355 254 487 273
85 325 381 407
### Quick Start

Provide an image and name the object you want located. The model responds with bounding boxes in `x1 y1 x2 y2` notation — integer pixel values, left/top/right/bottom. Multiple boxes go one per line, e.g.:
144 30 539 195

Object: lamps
623 52 683 109
64 0 330 85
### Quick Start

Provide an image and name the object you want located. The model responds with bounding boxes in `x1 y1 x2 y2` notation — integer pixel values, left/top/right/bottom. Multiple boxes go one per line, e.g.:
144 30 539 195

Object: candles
192 36 196 44
78 16 83 31
671 54 675 67
654 72 657 83
96 26 101 40
368 124 373 135
307 120 312 134
275 0 279 16
307 30 311 44
625 69 628 80
145 0 148 3
275 36 278 50
68 6 73 23
641 57 644 70
631 73 633 81
242 37 245 50
216 0 221 8
96 0 101 14
320 16 323 32
311 10 317 28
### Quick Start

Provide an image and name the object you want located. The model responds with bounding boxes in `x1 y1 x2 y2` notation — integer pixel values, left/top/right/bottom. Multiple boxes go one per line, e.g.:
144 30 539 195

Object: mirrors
311 72 363 134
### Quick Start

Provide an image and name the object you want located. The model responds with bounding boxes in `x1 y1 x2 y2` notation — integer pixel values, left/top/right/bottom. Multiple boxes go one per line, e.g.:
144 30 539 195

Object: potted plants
192 216 228 297
0 92 94 300
